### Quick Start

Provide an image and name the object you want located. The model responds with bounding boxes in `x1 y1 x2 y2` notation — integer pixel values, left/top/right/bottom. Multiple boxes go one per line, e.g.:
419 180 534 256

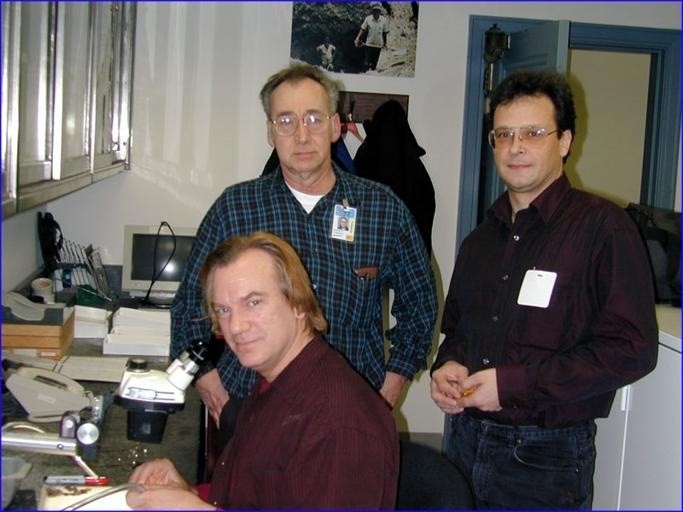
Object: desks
1 287 203 511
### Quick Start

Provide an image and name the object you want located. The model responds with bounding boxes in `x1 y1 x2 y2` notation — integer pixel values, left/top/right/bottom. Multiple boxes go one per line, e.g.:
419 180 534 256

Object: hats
369 1 382 10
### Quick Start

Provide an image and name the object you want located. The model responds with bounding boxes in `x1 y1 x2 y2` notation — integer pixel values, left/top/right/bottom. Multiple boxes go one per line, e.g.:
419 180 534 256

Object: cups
31 277 53 296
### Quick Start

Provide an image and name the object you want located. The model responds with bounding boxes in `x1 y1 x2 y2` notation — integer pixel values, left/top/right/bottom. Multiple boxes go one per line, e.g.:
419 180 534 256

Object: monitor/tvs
122 225 200 300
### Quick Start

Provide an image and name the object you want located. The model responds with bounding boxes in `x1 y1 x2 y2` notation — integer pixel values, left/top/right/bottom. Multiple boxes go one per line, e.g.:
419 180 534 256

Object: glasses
492 127 558 145
269 113 331 136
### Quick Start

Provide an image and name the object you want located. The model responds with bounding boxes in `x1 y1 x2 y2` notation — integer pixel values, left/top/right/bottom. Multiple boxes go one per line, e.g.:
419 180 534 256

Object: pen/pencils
43 457 108 485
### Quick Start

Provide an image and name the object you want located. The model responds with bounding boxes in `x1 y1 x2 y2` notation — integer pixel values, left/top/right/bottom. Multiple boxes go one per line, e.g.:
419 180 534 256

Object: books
52 231 172 358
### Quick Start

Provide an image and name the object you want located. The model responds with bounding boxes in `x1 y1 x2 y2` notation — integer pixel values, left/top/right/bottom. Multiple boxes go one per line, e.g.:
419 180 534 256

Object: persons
354 0 390 73
124 232 399 510
336 216 350 232
316 38 336 71
172 68 438 476
430 69 659 512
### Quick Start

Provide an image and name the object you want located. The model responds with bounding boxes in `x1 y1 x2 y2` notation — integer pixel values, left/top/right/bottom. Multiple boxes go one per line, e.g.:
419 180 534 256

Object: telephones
0 359 90 417
